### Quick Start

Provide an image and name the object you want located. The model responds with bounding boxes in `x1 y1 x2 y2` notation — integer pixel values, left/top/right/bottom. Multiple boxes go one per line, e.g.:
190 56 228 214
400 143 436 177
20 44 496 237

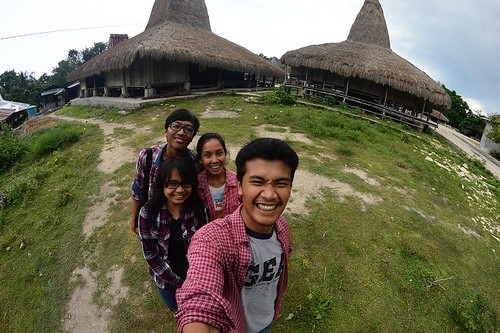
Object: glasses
168 123 198 134
162 180 193 189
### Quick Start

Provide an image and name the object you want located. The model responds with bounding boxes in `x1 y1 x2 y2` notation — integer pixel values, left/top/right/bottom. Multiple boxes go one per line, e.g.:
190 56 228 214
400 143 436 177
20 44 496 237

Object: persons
138 158 211 313
196 133 240 220
175 138 299 333
131 109 200 235
